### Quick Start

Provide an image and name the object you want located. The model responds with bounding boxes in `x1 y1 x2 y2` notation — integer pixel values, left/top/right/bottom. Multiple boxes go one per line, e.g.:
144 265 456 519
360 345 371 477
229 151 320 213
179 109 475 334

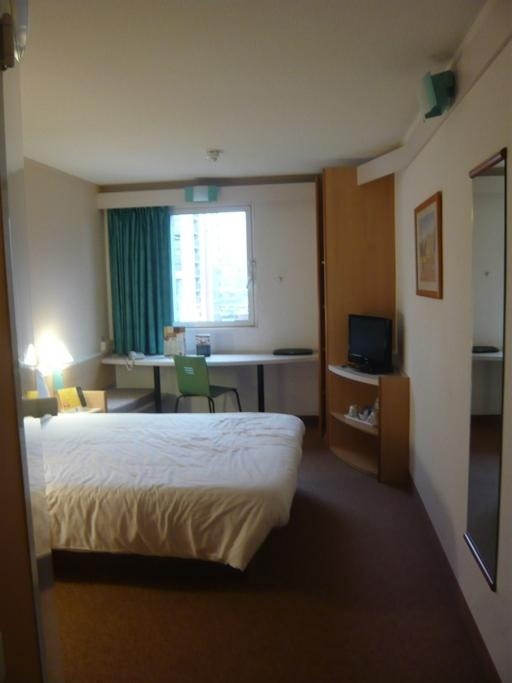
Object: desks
101 349 318 413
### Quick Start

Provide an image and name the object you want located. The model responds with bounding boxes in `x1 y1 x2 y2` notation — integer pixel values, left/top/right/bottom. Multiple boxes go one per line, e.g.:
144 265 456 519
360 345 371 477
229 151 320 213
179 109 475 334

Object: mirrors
461 146 507 592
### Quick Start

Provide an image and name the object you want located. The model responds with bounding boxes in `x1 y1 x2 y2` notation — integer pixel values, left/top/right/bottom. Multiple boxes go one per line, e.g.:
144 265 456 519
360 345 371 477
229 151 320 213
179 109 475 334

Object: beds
21 410 306 572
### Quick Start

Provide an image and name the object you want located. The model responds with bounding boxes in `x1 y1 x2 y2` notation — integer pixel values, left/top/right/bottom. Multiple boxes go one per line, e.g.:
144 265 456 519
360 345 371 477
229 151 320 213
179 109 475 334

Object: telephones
128 350 146 361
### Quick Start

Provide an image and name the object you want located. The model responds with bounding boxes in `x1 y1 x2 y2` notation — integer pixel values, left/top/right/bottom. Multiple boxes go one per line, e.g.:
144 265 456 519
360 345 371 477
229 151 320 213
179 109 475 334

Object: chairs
172 355 242 412
61 350 156 413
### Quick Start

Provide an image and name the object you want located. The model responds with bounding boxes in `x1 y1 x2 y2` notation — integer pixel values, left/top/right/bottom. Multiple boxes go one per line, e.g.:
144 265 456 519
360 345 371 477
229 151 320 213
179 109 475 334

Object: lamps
203 151 220 163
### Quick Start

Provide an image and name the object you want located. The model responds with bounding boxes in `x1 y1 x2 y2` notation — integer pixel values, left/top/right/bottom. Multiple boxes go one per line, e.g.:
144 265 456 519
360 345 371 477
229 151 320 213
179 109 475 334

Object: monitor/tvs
346 313 394 375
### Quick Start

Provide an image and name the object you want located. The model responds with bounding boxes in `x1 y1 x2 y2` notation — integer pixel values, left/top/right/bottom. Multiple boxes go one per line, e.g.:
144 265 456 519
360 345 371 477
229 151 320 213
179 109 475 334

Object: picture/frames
414 191 443 300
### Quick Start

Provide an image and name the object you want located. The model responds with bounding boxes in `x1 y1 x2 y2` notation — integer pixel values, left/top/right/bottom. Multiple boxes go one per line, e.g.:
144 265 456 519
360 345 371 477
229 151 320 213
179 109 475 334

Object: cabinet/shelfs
326 362 409 488
317 166 395 368
